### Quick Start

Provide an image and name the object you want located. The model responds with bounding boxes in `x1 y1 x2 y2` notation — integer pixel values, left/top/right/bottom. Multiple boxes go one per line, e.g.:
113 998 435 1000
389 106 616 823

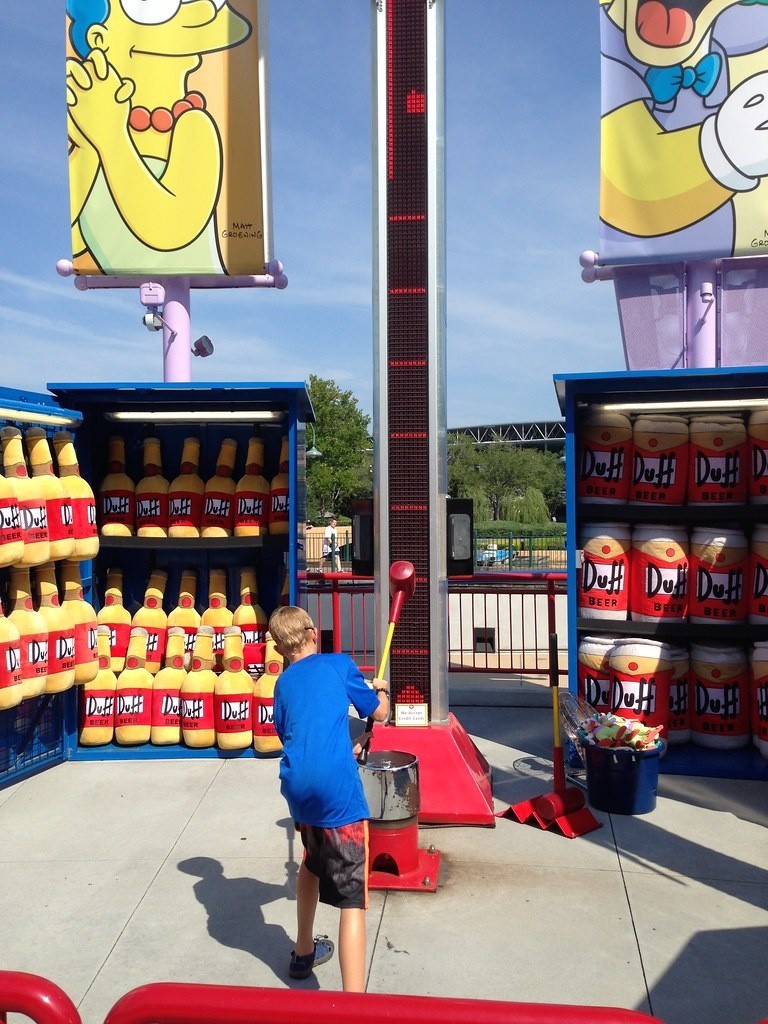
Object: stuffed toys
576 522 768 625
78 567 284 750
0 427 99 710
578 631 768 758
576 407 768 506
98 435 289 537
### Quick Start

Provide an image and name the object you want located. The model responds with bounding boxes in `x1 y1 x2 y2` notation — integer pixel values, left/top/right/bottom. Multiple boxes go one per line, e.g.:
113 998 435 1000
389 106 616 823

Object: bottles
152 626 188 745
214 626 255 750
253 631 284 753
80 624 117 745
35 562 75 694
101 437 135 536
115 627 154 744
0 597 22 710
180 625 219 748
0 426 100 568
135 435 290 538
60 559 99 685
97 566 267 674
8 568 48 699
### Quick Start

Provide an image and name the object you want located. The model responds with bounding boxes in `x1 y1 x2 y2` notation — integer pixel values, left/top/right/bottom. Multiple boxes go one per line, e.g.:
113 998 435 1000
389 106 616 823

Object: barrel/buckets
578 737 666 815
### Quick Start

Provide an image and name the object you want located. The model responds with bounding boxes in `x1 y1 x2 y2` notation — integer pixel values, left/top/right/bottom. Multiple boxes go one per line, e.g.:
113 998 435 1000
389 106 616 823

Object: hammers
357 559 416 767
531 631 584 818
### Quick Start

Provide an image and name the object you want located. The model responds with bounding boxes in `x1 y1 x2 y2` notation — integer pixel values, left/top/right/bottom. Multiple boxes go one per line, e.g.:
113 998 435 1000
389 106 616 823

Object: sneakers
289 934 334 980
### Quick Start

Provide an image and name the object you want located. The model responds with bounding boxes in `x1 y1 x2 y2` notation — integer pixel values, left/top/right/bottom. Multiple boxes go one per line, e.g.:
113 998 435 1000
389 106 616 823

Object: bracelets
376 688 389 700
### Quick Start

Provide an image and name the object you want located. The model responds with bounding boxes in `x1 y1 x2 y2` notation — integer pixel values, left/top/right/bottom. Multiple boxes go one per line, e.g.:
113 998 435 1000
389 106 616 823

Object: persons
317 517 344 573
268 606 390 993
